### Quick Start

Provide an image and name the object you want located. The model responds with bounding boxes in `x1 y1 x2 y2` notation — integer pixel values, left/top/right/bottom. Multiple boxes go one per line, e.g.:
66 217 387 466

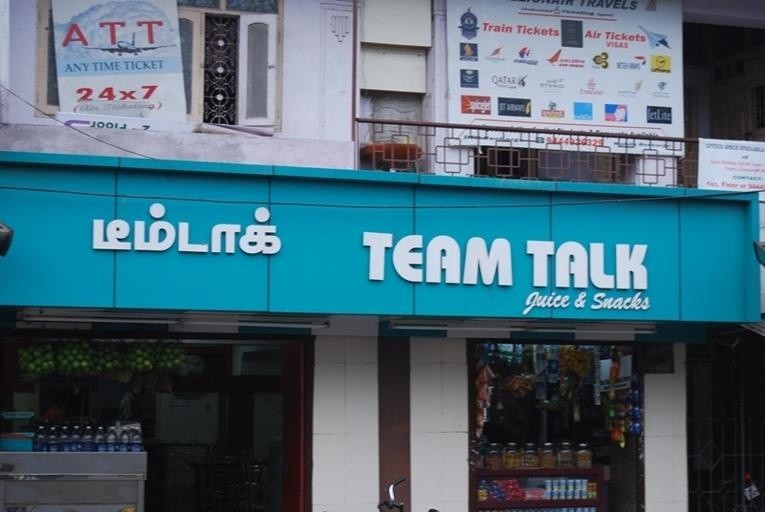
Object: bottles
476 479 488 502
478 507 597 512
485 441 594 470
607 405 616 419
609 365 619 382
545 478 597 499
608 386 616 402
32 423 143 452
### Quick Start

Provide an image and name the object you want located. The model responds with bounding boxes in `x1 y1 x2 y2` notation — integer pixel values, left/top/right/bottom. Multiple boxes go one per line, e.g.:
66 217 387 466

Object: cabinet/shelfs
469 464 607 512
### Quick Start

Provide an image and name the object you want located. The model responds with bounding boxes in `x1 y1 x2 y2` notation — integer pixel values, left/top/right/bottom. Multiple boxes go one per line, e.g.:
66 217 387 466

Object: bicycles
375 475 439 512
